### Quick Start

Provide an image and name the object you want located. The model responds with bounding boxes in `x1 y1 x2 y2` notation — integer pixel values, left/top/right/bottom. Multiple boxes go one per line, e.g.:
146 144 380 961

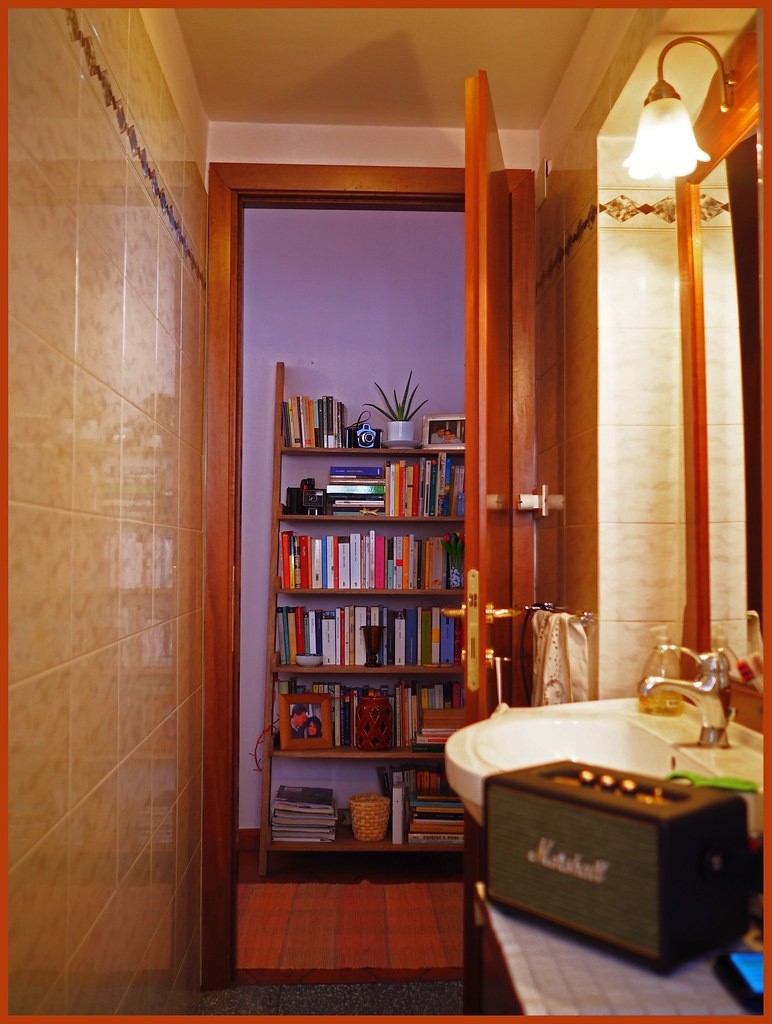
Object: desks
461 884 763 1016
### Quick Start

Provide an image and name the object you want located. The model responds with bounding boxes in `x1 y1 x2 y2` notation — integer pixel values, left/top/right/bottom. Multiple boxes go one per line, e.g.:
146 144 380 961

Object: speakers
482 761 752 974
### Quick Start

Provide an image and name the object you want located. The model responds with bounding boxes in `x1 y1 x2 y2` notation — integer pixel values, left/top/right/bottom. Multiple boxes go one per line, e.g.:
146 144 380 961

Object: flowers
440 530 465 572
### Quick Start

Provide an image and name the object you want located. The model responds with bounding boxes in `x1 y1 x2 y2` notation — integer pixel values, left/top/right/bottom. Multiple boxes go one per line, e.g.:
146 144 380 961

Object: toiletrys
636 625 683 716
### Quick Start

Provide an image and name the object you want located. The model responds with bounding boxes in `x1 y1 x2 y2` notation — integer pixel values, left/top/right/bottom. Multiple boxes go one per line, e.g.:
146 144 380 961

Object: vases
449 566 464 591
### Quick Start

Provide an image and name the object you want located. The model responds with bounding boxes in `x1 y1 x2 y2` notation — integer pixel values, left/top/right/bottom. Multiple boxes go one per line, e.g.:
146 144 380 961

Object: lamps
623 34 741 184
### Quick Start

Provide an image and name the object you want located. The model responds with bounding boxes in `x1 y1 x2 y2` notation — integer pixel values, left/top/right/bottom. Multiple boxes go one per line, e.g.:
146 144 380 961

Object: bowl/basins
295 655 322 668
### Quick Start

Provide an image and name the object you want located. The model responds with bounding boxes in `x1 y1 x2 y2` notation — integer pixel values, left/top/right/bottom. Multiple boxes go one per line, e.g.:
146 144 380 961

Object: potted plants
361 370 428 449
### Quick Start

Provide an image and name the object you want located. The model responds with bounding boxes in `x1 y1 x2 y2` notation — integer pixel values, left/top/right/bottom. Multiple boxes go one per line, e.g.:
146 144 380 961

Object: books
283 394 349 449
270 762 464 845
275 453 463 750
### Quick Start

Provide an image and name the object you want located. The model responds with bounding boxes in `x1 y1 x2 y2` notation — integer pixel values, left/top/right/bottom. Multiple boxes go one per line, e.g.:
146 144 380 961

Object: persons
430 426 461 443
291 703 307 738
306 716 323 738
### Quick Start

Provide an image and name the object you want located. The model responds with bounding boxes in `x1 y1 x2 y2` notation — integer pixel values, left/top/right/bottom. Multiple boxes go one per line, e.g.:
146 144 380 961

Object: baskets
349 793 390 842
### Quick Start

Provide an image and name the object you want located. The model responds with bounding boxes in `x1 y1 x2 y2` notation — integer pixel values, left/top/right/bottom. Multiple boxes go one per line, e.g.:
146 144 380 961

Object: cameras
346 423 383 450
286 487 326 516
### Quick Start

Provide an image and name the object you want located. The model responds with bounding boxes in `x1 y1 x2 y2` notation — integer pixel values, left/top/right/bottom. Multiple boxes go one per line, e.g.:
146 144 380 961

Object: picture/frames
277 692 334 751
421 413 465 450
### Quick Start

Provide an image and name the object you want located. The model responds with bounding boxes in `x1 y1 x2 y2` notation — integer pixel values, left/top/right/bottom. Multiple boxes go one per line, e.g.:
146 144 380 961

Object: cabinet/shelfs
257 361 469 879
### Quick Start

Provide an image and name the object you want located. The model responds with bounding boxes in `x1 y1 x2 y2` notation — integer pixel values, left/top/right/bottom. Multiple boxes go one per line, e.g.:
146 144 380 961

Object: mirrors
676 105 766 733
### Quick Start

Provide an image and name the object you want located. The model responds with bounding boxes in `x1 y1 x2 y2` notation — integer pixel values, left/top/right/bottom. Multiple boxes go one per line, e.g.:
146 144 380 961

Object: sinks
444 699 722 830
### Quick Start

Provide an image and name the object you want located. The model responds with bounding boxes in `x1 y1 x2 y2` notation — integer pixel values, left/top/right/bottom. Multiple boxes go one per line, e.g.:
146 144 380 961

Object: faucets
636 645 732 748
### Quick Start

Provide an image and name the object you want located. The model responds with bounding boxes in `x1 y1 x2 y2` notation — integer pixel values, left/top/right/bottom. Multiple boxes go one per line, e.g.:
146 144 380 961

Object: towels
531 609 588 707
